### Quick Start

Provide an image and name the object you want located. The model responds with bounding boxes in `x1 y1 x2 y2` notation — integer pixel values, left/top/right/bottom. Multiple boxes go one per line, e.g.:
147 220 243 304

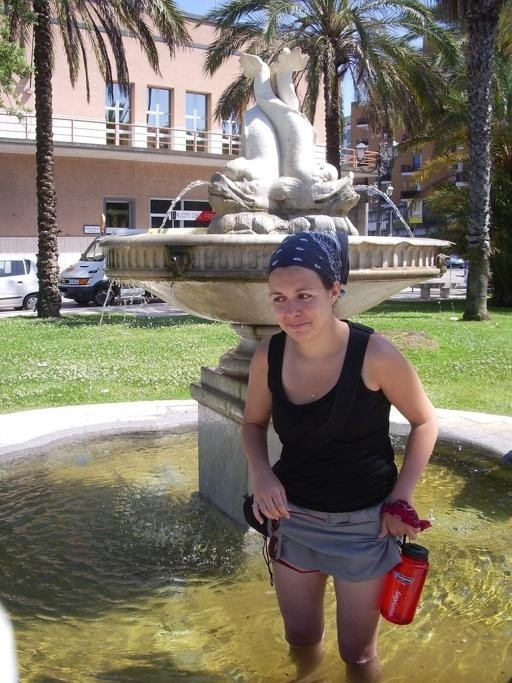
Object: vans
57 234 167 306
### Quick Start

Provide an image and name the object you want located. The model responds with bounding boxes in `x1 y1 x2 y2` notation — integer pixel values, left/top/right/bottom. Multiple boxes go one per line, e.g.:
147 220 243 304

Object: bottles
379 541 430 625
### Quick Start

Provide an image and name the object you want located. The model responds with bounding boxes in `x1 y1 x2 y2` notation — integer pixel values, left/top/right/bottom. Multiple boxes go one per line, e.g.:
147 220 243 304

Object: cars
445 253 466 269
0 252 39 311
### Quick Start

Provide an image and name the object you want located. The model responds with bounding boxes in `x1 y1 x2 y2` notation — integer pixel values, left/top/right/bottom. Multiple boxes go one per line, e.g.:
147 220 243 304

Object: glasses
268 510 326 573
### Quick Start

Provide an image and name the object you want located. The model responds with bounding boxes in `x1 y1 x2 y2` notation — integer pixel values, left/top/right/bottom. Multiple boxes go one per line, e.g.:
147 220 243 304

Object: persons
241 232 439 682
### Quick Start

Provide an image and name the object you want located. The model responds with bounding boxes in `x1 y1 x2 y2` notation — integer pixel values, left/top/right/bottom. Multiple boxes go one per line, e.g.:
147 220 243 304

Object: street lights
386 182 394 236
354 121 399 236
